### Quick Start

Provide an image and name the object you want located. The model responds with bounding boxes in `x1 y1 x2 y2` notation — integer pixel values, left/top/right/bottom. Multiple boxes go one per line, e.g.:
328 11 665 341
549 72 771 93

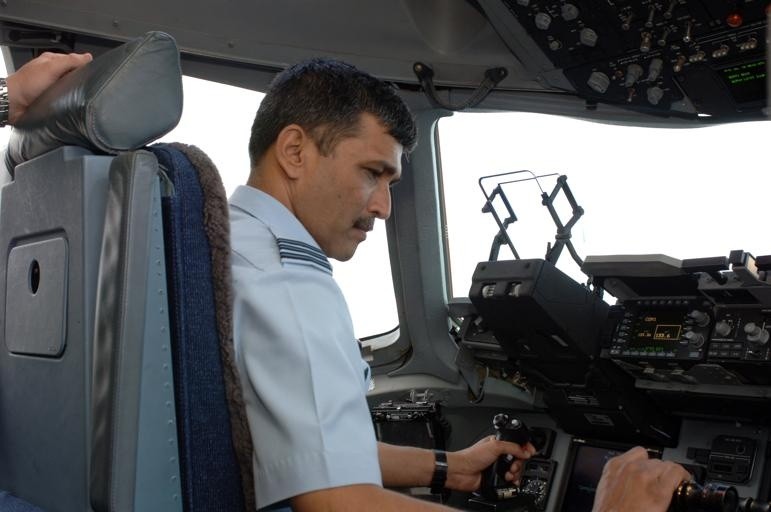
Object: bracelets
0 78 9 128
429 449 448 494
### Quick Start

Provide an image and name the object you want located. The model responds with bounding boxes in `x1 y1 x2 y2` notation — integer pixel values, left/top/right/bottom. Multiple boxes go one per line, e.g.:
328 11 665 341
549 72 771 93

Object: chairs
0 32 278 512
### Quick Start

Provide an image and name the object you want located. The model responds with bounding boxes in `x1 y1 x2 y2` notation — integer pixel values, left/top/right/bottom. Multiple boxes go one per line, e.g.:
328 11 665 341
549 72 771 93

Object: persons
0 51 94 126
225 58 692 512
